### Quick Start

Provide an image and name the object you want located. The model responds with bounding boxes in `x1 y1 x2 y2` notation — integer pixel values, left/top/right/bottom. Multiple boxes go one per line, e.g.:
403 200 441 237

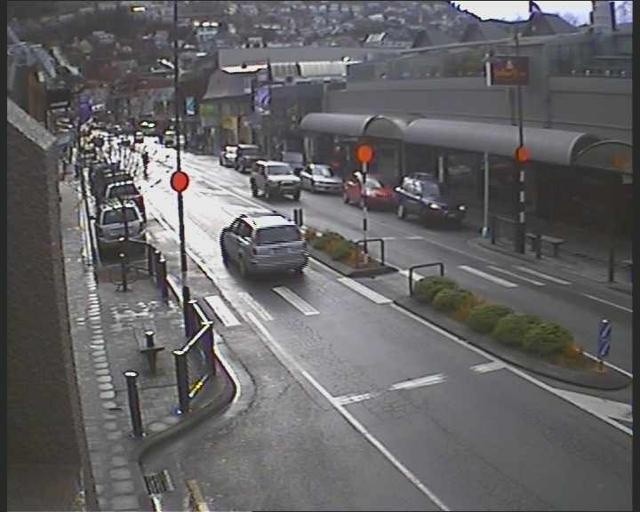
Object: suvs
218 142 302 202
218 210 309 279
393 170 469 231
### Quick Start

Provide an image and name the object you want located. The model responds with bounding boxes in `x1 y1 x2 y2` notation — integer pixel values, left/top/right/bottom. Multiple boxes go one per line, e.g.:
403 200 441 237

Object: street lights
155 18 223 339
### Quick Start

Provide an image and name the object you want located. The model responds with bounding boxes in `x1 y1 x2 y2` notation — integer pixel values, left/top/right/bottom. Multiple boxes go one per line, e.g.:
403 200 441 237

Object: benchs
523 229 567 258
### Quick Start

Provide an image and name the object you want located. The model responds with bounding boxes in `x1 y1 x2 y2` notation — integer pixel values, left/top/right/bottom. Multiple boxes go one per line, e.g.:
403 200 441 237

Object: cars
371 54 634 80
75 117 178 265
298 162 345 195
342 175 395 210
